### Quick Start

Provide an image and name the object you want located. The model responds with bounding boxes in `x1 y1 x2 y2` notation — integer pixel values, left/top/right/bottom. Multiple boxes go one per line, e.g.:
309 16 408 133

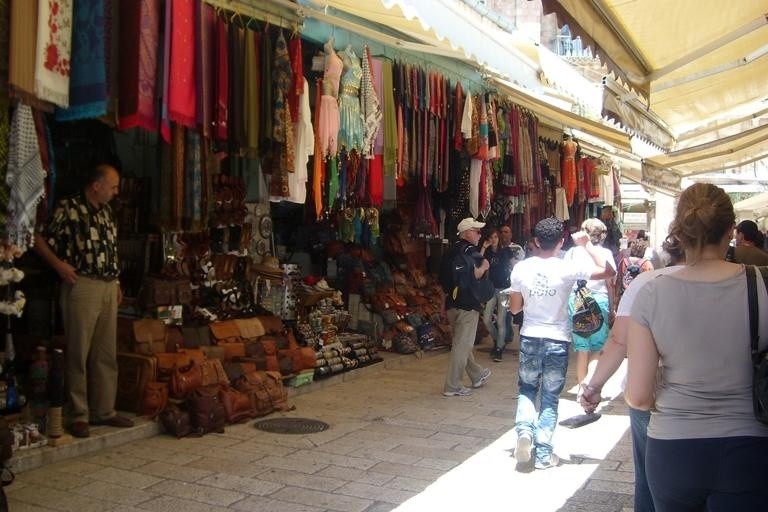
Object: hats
735 220 758 236
457 218 486 232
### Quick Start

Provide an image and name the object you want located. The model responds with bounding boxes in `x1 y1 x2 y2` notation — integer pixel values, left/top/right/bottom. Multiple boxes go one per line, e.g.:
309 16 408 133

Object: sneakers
535 453 559 469
514 432 531 462
443 386 473 396
472 368 491 387
490 339 505 362
577 386 585 402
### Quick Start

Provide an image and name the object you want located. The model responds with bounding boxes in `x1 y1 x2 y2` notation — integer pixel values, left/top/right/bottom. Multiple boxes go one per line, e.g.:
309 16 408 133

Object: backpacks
623 258 648 289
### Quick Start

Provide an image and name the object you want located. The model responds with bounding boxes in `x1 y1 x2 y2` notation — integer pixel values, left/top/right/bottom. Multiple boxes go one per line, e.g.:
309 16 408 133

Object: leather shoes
68 422 91 438
92 414 135 427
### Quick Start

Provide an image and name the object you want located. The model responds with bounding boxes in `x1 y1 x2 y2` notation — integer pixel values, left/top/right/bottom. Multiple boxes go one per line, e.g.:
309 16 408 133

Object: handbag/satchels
445 243 494 306
117 316 316 440
752 348 767 423
330 236 452 354
571 280 604 338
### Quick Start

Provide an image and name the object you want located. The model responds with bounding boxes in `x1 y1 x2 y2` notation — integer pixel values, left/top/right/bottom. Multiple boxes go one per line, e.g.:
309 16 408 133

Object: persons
562 135 580 204
313 34 345 159
581 217 686 512
439 213 663 403
507 216 617 469
31 162 133 438
626 181 768 511
337 45 365 157
726 218 768 267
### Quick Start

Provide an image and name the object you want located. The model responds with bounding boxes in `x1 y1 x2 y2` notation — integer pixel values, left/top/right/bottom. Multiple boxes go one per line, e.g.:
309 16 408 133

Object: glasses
471 230 481 234
